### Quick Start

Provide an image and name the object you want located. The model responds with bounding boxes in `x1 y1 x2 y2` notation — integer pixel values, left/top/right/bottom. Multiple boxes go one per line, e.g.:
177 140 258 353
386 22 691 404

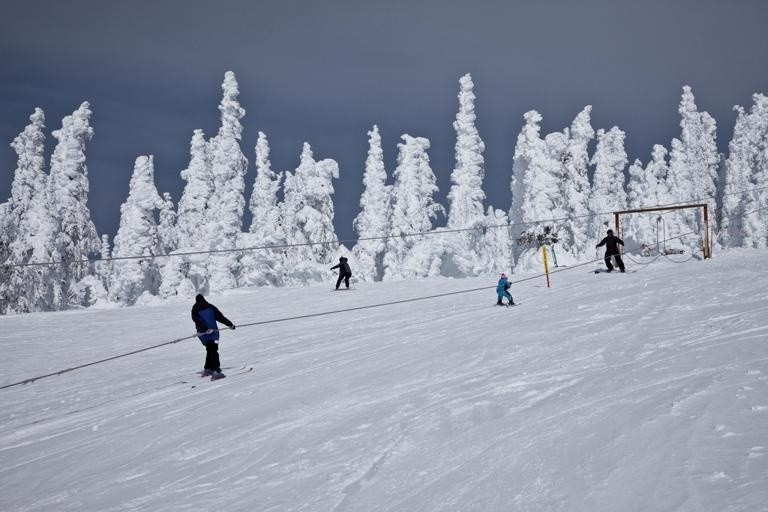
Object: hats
501 273 508 279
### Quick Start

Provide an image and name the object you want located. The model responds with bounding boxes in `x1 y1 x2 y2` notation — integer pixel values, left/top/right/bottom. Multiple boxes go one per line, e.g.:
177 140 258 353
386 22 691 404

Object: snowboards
595 268 636 273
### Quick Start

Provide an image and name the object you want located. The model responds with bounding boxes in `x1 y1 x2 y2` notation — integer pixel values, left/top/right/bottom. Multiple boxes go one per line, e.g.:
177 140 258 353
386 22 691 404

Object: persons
191 294 235 380
330 256 352 290
496 272 514 306
595 230 626 273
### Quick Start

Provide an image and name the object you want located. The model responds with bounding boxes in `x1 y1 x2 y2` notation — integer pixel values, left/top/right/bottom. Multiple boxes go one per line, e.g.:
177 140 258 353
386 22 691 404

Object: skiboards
182 365 253 388
329 287 355 291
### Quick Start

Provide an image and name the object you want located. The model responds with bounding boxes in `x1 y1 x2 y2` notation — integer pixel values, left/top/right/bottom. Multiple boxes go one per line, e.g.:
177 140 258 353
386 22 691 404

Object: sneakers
508 301 514 305
211 370 225 381
497 300 503 305
202 368 213 376
